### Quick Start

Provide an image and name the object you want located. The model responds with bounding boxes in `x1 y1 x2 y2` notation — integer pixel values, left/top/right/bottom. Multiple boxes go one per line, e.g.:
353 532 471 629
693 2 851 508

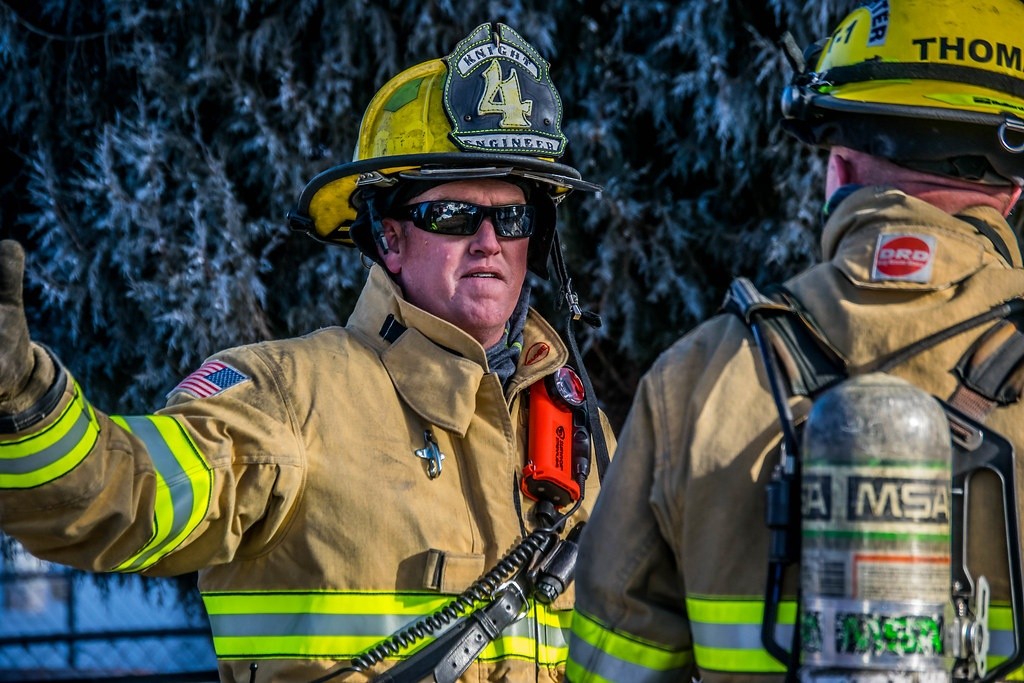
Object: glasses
388 200 534 238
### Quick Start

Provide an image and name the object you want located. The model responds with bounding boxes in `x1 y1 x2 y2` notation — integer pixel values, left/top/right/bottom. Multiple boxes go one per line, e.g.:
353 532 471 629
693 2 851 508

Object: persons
0 21 619 683
562 0 1024 683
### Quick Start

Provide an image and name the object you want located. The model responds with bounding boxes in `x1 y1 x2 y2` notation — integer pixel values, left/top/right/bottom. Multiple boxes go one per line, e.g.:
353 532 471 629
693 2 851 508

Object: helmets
781 0 1024 153
286 22 581 247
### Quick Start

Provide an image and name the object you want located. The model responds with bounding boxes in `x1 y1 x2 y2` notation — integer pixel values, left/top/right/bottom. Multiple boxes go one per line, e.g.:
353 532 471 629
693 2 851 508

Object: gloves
0 240 57 419
